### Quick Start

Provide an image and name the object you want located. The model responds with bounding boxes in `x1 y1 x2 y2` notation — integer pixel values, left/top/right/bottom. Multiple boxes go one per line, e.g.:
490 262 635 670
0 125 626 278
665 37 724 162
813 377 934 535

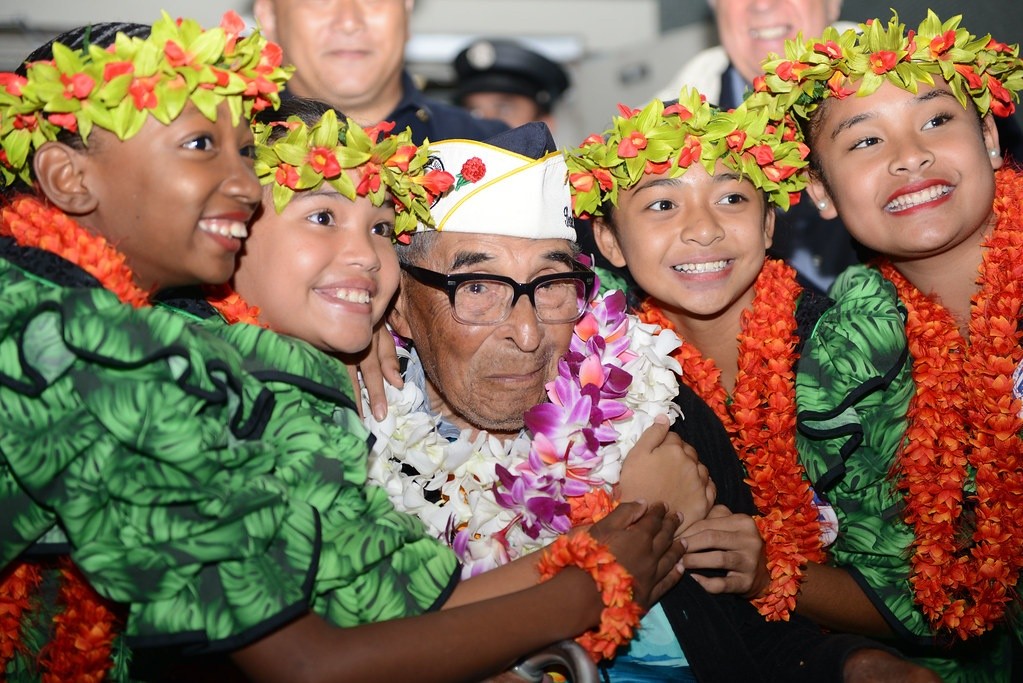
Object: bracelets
537 538 642 660
751 510 806 621
565 487 622 526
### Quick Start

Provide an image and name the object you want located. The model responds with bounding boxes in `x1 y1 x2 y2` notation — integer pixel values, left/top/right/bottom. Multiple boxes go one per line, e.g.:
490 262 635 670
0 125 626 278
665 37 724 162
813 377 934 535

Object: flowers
565 7 1023 219
0 9 454 244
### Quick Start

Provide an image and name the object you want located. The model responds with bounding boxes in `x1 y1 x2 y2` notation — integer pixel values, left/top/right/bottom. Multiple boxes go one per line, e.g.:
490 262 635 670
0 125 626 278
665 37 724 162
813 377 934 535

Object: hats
395 122 579 240
444 38 572 116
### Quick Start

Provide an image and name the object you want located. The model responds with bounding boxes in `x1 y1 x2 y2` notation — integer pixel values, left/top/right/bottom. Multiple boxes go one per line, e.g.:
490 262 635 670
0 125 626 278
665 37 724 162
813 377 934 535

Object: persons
345 87 1023 683
348 142 941 683
112 98 718 683
663 0 872 285
0 14 687 683
246 0 558 158
452 38 570 130
737 15 1023 645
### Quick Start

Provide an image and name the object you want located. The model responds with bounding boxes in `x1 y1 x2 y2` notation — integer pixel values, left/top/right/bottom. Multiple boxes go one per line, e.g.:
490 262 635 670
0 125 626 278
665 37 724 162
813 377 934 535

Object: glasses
398 254 597 327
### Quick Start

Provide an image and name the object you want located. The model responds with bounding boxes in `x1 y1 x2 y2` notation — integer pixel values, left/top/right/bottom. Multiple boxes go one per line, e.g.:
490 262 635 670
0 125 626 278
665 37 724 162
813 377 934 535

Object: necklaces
344 292 683 578
880 166 1022 635
205 285 268 330
0 191 151 308
630 260 829 567
